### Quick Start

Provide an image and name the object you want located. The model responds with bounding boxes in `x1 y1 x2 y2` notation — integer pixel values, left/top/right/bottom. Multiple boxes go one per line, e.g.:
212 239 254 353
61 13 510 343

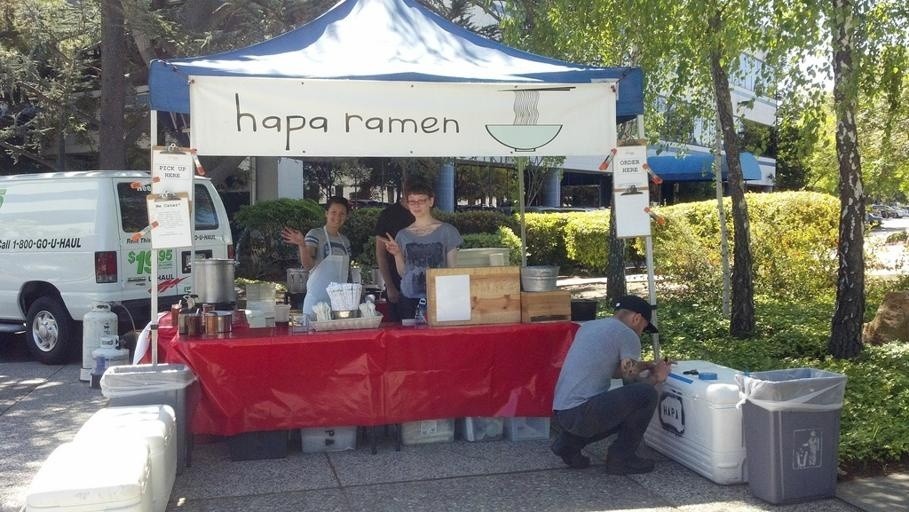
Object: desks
375 320 579 451
135 310 175 365
163 325 385 457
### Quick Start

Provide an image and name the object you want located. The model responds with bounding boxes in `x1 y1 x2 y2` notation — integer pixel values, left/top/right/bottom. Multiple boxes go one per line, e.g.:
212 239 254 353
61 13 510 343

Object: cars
863 201 909 224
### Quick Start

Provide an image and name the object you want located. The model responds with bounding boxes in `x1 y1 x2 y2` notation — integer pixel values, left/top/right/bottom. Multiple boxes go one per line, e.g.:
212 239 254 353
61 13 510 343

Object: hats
614 296 658 333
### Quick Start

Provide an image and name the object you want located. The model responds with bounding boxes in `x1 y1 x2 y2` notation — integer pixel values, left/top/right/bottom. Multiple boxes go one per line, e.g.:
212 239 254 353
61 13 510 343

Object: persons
380 182 463 321
376 178 431 322
550 296 671 474
281 196 353 316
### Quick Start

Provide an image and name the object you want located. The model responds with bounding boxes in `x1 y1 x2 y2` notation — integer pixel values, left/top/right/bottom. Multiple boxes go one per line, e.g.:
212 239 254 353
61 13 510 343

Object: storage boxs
76 402 179 512
390 416 458 445
296 422 358 454
570 298 599 321
458 416 506 443
641 357 756 487
518 288 574 326
420 266 522 324
226 428 292 461
23 439 158 512
504 416 551 441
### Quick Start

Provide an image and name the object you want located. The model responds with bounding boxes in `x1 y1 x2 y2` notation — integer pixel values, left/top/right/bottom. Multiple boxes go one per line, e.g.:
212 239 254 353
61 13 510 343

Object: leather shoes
551 439 589 467
607 457 654 474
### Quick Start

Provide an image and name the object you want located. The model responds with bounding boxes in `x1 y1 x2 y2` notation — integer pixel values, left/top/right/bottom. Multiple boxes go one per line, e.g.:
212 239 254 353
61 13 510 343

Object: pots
371 266 384 285
285 268 309 293
187 258 241 306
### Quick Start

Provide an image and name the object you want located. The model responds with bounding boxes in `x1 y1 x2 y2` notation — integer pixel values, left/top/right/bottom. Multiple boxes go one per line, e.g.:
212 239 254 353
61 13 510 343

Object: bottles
205 312 218 338
265 317 277 333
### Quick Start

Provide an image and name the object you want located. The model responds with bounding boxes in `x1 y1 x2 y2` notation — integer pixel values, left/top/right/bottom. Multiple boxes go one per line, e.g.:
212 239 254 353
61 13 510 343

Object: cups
202 304 215 312
293 314 307 334
274 305 291 325
170 304 183 328
184 314 203 338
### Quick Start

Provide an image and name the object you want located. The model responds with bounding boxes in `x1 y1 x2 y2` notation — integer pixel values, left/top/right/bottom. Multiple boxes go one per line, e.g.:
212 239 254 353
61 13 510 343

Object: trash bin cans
734 368 848 504
99 363 198 476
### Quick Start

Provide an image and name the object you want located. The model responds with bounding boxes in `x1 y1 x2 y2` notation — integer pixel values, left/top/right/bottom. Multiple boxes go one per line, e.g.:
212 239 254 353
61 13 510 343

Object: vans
0 168 234 362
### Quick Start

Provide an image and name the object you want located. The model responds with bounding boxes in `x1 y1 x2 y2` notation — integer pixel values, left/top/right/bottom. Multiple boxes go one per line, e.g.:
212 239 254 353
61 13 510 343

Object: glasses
407 199 426 205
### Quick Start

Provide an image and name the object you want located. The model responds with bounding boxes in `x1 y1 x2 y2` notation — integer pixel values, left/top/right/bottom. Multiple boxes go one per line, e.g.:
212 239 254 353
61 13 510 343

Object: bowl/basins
485 123 564 152
330 310 359 319
